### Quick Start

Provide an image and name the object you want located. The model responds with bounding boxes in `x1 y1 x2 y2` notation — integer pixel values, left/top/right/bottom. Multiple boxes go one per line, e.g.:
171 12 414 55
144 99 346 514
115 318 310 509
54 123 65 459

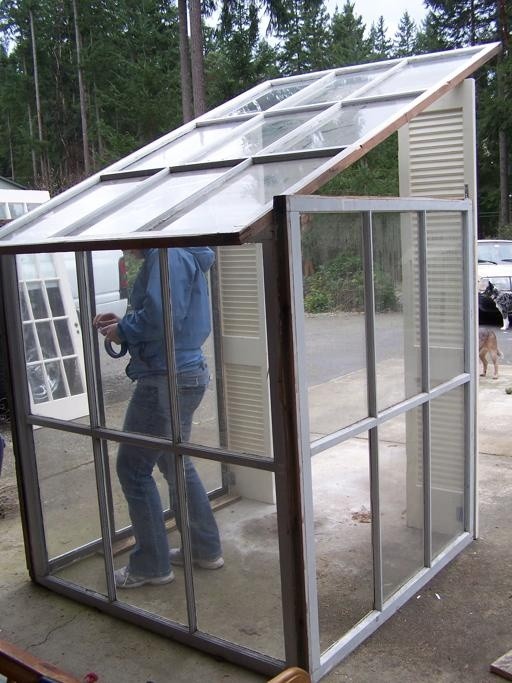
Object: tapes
104 336 127 358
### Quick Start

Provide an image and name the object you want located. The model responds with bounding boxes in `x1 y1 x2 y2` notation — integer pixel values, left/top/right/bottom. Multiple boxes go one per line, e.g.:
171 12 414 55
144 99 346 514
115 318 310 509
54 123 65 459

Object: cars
475 238 511 322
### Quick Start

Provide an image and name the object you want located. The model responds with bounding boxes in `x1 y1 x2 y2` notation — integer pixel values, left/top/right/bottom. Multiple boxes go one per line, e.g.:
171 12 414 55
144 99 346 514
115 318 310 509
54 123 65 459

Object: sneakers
115 565 175 588
169 547 225 569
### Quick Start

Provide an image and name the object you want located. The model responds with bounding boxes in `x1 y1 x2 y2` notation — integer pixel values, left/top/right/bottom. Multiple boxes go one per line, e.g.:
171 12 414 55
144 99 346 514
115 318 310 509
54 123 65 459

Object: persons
94 236 226 589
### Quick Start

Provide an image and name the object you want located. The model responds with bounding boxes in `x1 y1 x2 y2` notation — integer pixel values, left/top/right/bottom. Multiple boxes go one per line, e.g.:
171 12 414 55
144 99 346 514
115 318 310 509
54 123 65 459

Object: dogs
479 329 504 379
480 281 512 331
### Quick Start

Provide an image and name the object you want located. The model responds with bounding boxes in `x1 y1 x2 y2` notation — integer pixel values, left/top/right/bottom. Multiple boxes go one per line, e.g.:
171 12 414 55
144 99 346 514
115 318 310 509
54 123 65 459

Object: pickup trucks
1 247 130 408
